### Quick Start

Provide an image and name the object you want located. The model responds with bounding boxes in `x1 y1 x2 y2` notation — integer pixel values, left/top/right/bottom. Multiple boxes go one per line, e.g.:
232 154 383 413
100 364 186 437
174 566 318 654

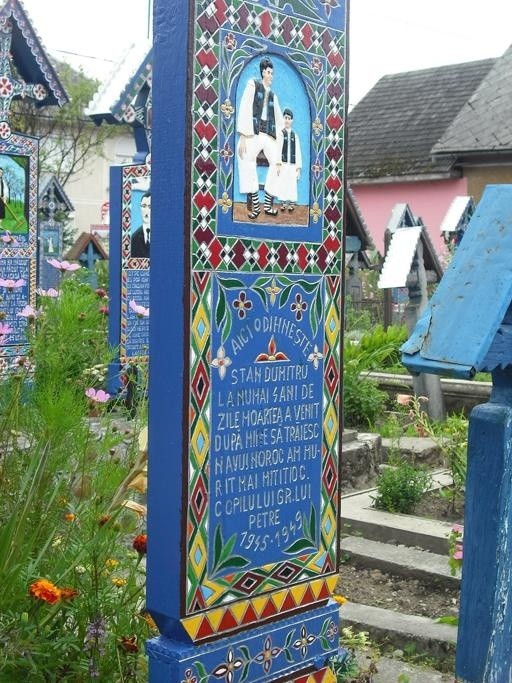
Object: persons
276 109 302 212
131 192 151 259
237 56 285 220
0 168 7 224
